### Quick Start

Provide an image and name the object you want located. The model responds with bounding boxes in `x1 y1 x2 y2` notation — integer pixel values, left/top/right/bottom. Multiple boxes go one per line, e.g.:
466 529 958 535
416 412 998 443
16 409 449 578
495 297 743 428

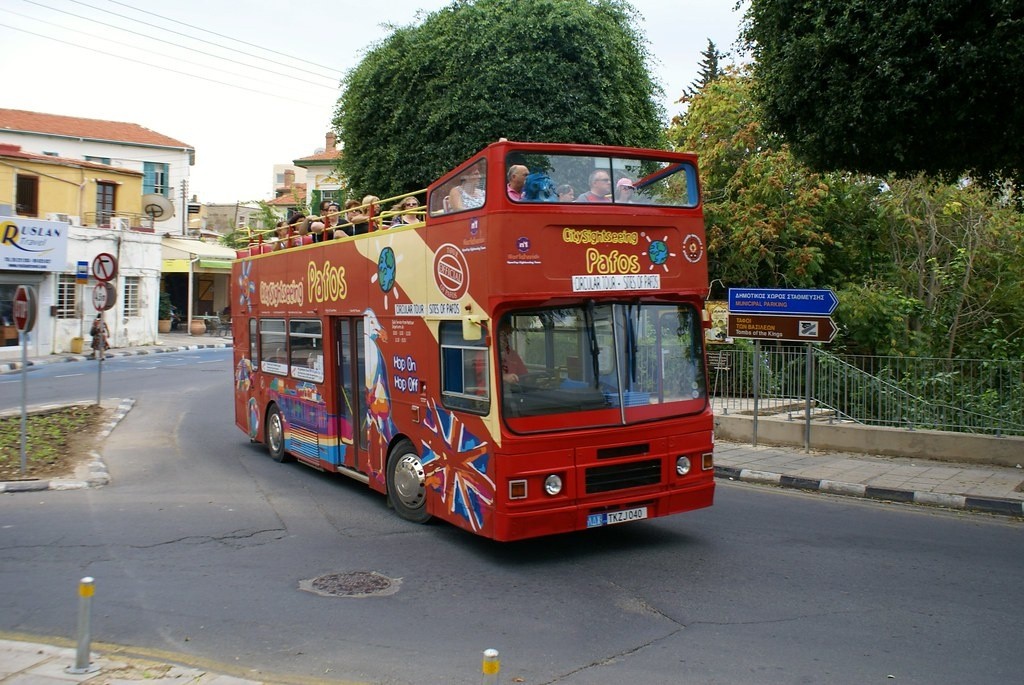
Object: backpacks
521 166 559 202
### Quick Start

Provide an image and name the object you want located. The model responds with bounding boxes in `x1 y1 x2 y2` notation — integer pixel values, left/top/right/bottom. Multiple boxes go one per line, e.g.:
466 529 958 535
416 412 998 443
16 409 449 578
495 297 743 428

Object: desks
192 315 220 332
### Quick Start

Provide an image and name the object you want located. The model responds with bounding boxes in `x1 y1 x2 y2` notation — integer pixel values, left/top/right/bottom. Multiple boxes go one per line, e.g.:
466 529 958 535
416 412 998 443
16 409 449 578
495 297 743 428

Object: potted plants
159 292 172 333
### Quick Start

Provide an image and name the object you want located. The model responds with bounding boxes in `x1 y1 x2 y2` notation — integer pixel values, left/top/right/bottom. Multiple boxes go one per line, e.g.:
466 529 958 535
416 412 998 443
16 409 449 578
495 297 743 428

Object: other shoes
91 353 96 356
101 356 105 361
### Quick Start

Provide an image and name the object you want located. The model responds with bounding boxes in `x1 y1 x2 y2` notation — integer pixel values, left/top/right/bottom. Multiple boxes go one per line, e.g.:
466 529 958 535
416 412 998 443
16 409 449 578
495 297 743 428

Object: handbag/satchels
90 326 99 336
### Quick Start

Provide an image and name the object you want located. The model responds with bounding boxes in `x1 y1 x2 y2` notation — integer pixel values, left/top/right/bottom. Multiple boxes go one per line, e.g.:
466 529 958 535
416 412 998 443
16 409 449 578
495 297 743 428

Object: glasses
329 202 340 206
403 202 418 206
321 208 329 211
593 178 611 183
621 185 633 190
349 209 361 212
468 172 480 175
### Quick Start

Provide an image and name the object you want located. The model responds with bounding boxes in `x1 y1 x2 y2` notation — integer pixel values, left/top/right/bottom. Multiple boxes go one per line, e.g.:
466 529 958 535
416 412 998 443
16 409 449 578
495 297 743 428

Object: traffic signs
728 288 841 315
727 314 840 343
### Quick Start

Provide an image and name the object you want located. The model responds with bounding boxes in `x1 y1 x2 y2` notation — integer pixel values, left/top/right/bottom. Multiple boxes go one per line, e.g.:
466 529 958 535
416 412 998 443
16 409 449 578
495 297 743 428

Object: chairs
234 224 392 259
207 315 232 336
276 345 322 368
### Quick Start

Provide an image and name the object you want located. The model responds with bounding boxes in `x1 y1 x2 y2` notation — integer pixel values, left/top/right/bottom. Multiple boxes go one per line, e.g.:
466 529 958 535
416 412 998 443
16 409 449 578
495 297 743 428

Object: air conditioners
45 212 81 227
109 217 130 232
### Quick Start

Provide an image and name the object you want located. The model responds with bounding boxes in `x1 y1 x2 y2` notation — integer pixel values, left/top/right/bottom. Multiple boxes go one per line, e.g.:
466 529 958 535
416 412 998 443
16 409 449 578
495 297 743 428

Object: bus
226 141 716 542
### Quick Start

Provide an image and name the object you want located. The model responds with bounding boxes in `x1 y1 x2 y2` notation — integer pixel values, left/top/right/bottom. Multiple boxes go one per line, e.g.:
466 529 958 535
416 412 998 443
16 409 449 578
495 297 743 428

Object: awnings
161 237 237 274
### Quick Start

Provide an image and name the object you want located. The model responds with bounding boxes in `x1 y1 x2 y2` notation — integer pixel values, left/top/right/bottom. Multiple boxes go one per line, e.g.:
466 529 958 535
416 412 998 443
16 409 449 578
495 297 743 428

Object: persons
507 164 530 203
449 161 485 210
475 325 528 398
90 313 110 361
169 303 181 331
268 194 381 253
557 183 575 201
613 178 637 204
390 195 422 227
575 169 613 203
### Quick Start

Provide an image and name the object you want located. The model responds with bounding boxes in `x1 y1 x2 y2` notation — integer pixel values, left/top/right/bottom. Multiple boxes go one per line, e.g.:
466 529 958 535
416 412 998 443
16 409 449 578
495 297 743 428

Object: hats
617 177 633 188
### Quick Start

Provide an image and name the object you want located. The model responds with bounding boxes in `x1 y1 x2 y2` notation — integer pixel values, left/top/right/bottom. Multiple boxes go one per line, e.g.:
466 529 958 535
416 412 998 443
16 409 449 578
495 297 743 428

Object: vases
190 319 206 337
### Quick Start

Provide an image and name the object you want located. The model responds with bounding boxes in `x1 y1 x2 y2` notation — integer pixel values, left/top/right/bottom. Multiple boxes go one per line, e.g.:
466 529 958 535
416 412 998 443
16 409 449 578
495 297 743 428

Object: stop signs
13 284 36 333
